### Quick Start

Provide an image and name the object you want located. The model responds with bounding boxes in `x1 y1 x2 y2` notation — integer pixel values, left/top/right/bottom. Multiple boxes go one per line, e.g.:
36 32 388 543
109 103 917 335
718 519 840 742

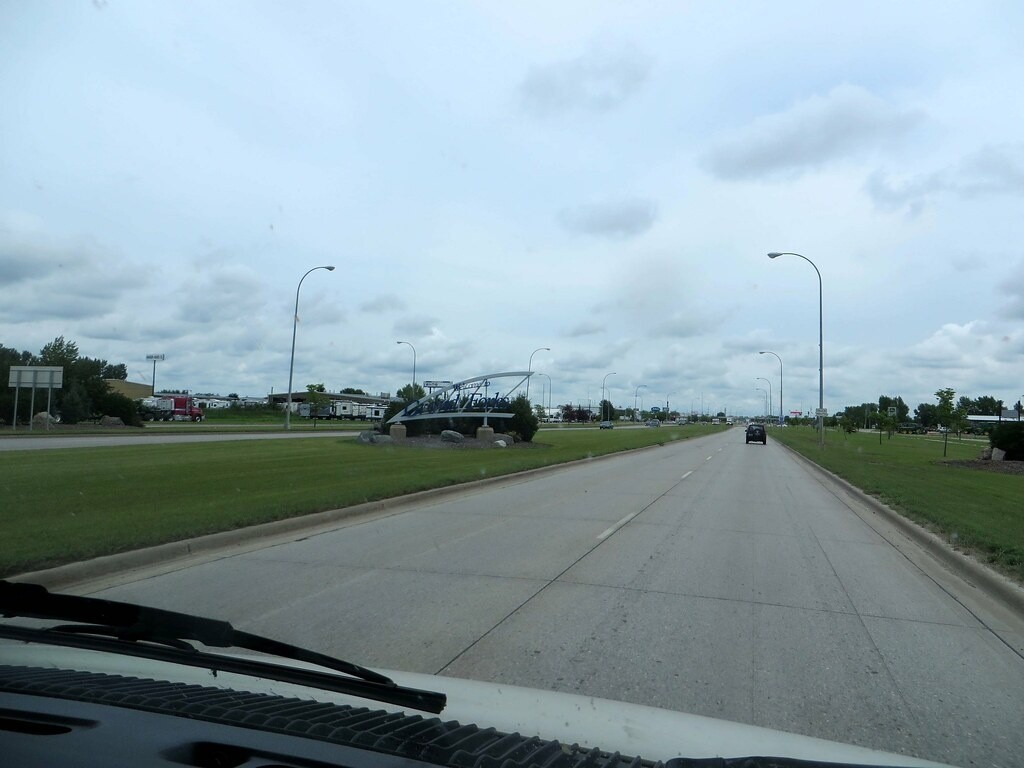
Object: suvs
744 424 766 445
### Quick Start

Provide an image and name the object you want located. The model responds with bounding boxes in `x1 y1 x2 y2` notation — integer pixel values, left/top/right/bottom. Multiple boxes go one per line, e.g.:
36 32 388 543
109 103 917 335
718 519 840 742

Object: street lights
397 341 416 399
760 350 783 426
767 250 824 449
601 387 611 421
757 378 772 416
666 391 678 413
285 265 335 426
634 384 648 422
601 372 617 422
527 348 550 401
539 373 552 423
691 397 699 414
756 388 768 416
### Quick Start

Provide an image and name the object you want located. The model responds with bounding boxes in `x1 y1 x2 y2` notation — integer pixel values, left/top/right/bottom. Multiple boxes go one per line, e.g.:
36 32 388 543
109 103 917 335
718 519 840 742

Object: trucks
726 417 734 425
712 418 720 425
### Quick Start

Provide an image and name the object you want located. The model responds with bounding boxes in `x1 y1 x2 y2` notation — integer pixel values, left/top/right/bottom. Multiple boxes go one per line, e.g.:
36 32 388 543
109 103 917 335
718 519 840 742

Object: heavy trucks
132 395 205 423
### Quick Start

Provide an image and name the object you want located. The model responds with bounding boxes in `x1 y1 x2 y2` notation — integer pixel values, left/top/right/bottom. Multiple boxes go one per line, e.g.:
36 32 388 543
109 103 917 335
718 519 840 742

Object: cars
599 421 614 429
645 421 652 426
650 419 661 428
670 419 708 424
899 422 955 435
541 415 591 424
679 420 687 426
